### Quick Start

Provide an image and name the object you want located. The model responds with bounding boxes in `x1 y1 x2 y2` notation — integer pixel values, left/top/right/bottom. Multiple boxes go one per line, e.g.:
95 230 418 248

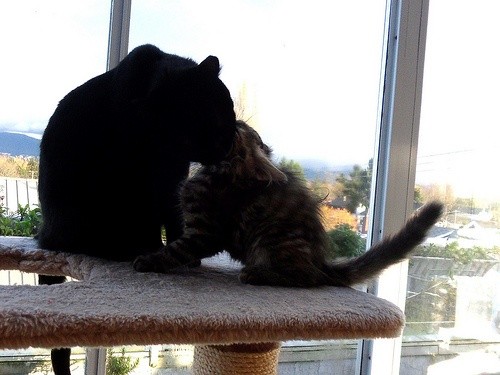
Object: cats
33 43 238 268
135 121 446 289
